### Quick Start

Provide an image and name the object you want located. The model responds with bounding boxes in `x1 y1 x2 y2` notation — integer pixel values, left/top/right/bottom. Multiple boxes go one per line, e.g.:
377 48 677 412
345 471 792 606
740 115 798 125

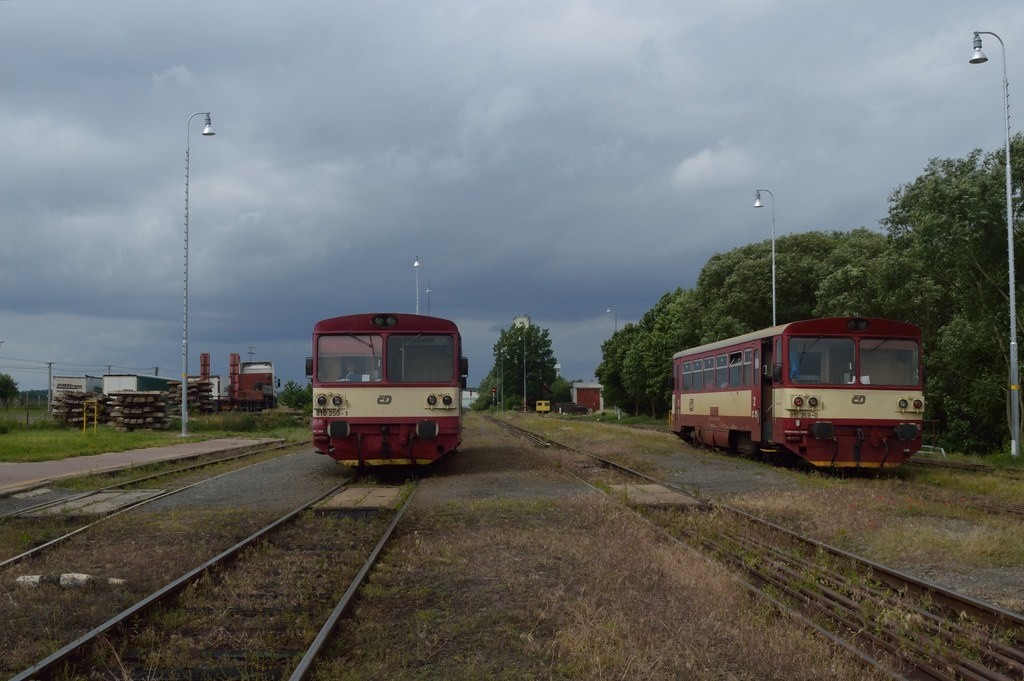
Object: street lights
519 335 527 413
182 112 216 436
753 190 776 327
607 305 617 413
414 255 420 315
426 285 432 316
970 31 1020 457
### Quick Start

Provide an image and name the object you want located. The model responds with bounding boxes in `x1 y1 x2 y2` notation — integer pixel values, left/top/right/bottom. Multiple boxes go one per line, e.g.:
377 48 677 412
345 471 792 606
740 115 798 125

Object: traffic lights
492 388 495 392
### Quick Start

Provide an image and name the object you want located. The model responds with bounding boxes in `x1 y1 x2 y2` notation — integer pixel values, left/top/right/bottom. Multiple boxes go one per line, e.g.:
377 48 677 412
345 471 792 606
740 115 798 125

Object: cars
553 402 588 414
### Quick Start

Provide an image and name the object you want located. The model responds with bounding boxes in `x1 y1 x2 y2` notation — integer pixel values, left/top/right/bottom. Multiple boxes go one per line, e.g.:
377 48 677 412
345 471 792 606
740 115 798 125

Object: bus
672 317 924 469
305 312 468 468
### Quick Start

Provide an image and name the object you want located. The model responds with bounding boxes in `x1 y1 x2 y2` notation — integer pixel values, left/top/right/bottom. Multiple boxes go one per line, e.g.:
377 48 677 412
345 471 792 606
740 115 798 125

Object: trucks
199 353 281 412
